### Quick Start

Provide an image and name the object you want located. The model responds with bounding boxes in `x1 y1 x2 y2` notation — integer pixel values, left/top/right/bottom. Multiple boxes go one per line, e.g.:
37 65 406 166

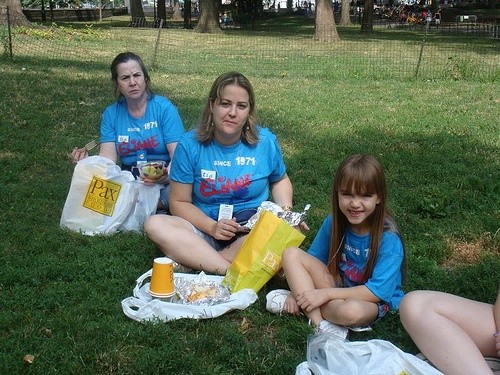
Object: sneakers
265 289 291 316
318 319 347 339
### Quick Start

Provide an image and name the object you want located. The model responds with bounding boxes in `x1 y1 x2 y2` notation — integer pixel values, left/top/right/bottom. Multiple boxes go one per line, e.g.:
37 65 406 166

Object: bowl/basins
137 160 166 180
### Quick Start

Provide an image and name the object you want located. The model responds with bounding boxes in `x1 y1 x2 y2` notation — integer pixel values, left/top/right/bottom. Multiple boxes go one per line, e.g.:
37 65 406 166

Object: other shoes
164 255 192 273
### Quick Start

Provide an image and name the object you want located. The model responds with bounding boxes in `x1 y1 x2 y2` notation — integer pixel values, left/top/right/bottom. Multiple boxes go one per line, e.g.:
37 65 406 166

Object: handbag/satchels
219 210 307 295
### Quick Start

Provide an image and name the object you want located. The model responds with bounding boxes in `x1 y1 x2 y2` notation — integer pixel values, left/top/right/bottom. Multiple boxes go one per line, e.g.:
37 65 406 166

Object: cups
148 257 176 298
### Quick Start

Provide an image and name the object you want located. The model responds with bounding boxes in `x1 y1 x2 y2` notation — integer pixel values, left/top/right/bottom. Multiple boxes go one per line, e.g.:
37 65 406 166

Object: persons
399 285 500 375
264 153 406 360
70 52 186 214
144 71 310 279
23 0 500 32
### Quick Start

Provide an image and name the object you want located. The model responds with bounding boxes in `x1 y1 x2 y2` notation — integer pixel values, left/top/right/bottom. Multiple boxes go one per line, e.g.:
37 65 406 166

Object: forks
70 141 98 157
